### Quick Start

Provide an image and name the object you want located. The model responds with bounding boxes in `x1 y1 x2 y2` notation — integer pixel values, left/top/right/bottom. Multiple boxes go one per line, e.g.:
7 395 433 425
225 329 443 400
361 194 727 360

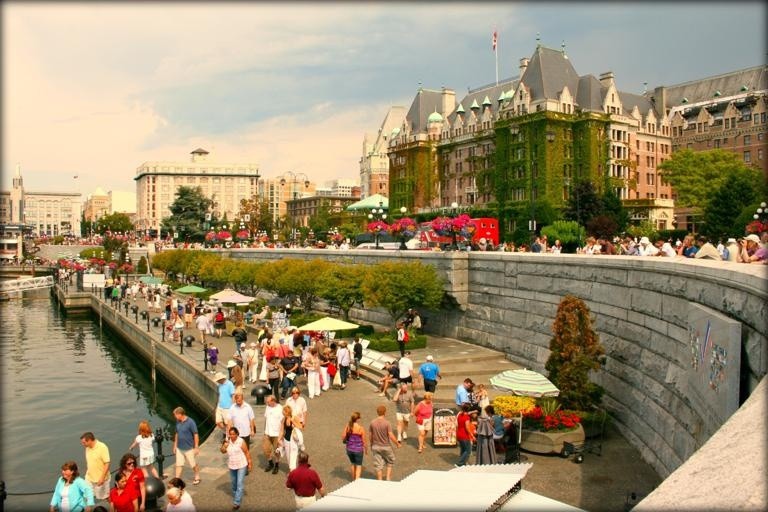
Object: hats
743 232 765 248
226 359 238 369
426 355 434 361
250 343 256 347
211 371 227 383
639 236 651 245
727 237 737 243
240 343 245 348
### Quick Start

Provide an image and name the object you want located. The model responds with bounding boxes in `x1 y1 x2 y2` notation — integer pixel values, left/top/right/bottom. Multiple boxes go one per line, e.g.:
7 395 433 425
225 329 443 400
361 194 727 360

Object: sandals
192 479 201 485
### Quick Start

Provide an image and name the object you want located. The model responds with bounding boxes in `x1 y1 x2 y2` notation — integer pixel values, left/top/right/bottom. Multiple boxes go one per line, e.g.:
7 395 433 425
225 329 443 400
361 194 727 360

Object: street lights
211 221 245 233
71 174 95 247
752 201 767 250
256 229 268 248
288 226 341 249
446 201 462 252
279 170 311 250
90 250 130 291
398 205 410 249
368 207 387 250
23 210 27 225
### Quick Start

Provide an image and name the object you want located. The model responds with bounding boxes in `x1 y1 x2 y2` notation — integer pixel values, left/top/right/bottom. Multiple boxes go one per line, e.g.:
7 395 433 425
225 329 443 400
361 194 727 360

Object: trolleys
560 439 604 465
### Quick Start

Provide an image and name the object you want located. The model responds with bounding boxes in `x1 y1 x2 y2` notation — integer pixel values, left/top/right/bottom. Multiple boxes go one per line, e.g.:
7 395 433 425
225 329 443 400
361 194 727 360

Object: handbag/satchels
235 349 243 370
326 359 337 378
342 425 352 444
247 356 252 365
424 422 433 433
331 369 342 386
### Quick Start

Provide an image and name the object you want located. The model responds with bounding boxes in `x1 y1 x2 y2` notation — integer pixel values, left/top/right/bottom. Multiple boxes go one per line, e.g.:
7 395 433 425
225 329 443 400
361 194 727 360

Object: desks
225 321 251 336
433 408 457 447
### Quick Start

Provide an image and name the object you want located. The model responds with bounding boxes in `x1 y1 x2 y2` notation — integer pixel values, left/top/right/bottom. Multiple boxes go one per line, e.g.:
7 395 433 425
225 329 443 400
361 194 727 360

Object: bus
417 217 500 253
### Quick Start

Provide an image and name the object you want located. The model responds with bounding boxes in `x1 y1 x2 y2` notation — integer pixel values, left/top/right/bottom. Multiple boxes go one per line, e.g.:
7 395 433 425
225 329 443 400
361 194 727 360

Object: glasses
127 461 138 466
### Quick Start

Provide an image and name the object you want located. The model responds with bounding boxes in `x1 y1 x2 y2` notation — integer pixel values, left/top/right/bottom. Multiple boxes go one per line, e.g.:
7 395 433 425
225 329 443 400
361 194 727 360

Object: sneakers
397 433 401 442
374 388 385 397
418 448 422 453
232 504 240 509
402 431 408 440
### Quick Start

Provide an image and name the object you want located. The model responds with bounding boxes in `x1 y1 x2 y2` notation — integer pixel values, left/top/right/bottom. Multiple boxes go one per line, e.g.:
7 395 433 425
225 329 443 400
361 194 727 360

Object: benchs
404 313 429 335
324 302 342 317
261 319 272 327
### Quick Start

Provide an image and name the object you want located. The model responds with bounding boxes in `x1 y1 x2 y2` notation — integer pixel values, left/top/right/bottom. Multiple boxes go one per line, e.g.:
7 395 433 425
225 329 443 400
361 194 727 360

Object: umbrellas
489 368 560 445
347 191 389 224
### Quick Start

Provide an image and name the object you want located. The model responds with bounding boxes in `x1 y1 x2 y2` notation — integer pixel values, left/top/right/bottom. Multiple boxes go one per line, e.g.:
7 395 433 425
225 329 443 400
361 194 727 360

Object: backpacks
216 312 224 322
265 345 275 362
403 330 409 343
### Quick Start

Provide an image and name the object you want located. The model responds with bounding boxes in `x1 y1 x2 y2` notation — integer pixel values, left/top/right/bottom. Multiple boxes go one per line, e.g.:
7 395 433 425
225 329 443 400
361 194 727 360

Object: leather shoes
264 464 274 472
271 467 279 474
